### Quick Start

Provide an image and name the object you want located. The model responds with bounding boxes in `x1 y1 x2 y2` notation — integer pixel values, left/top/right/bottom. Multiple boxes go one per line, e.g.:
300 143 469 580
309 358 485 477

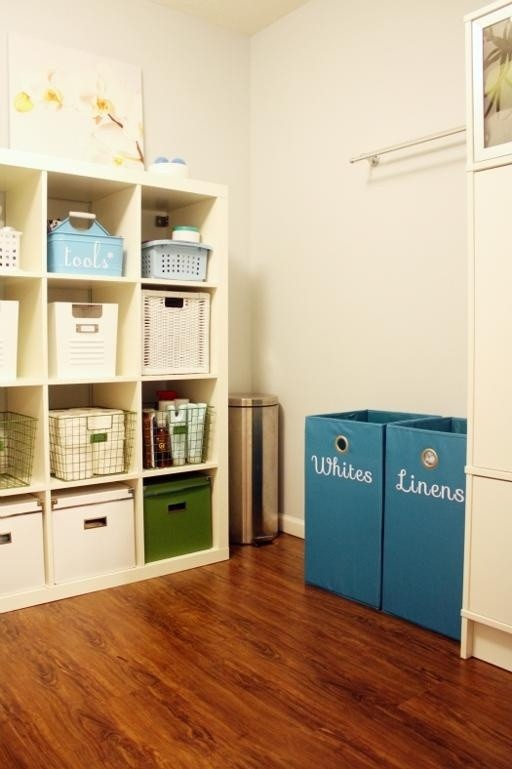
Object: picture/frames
463 0 512 173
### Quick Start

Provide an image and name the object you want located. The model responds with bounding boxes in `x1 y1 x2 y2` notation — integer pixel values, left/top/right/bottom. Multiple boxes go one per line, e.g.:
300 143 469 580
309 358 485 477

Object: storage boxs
47 301 118 376
48 211 124 277
381 416 469 643
143 474 212 565
305 408 440 612
0 300 19 380
51 481 136 586
0 493 46 595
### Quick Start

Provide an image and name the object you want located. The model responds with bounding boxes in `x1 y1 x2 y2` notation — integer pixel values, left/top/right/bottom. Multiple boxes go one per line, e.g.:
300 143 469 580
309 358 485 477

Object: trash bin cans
229 393 280 546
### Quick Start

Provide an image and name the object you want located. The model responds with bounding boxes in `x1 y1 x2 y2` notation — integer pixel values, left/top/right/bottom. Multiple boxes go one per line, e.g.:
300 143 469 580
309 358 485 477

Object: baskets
0 217 216 490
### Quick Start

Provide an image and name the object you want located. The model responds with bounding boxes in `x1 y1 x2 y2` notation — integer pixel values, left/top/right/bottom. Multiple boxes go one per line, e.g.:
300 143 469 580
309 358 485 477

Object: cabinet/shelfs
0 146 230 615
460 166 512 673
